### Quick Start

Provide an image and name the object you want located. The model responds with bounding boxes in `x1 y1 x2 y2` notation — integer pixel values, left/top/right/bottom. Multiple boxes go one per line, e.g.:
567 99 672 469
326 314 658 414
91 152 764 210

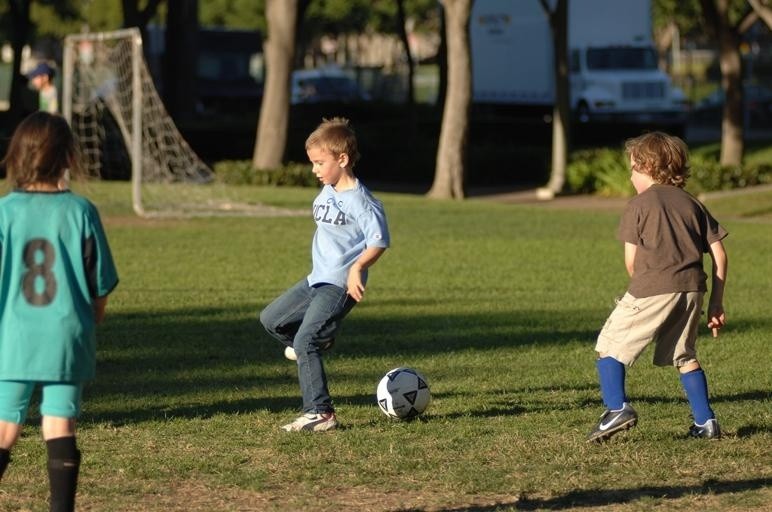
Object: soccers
376 367 430 418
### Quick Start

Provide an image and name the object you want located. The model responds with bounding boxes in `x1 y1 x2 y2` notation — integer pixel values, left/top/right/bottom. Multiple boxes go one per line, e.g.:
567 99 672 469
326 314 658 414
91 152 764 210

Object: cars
291 68 371 108
696 87 771 133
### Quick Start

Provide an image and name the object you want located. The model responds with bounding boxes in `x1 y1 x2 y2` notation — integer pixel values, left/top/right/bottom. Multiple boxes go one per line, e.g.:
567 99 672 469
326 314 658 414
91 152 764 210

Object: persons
30 63 59 115
0 110 121 512
580 130 731 442
259 114 390 433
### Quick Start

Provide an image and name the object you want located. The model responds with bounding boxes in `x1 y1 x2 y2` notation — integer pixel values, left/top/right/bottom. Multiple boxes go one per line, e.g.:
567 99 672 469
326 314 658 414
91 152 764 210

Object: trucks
470 0 689 132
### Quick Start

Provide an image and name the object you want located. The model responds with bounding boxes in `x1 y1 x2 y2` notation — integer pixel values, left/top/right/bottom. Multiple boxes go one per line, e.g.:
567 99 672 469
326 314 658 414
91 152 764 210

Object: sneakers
583 403 638 444
673 419 722 441
284 340 331 361
279 410 338 434
